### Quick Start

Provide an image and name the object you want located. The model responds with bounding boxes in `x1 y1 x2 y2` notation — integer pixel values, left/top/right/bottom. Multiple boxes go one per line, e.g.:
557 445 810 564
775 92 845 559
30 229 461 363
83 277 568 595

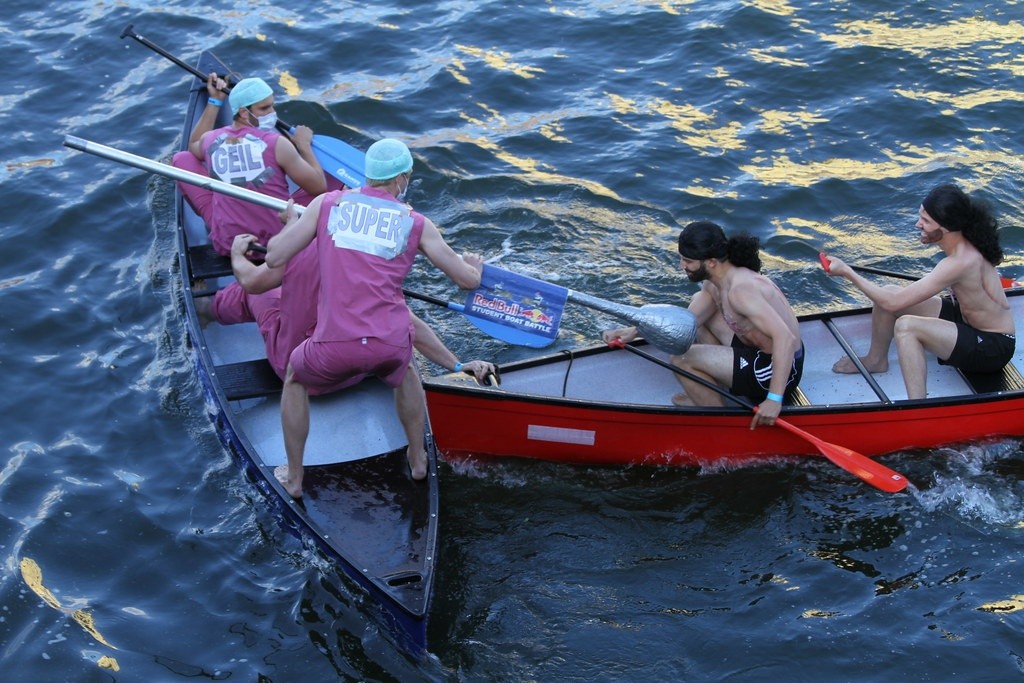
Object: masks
397 174 409 200
245 107 277 130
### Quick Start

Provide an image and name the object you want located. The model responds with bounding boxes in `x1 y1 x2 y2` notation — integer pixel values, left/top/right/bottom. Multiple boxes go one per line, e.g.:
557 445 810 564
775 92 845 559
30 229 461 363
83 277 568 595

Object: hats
228 77 273 116
365 139 414 181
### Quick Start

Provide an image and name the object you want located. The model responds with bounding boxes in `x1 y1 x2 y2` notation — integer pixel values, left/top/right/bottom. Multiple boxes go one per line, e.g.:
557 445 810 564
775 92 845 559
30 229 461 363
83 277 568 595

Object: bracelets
767 392 783 402
453 362 463 372
207 97 224 106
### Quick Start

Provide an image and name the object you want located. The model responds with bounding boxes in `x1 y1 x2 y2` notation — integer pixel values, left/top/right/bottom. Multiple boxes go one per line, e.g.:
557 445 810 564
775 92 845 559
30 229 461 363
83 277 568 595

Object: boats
175 51 440 655
422 286 1024 468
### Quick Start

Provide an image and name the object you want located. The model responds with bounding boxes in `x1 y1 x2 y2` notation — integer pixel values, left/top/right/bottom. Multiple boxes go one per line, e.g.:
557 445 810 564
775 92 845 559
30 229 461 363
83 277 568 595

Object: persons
825 182 1016 400
602 220 805 430
172 72 495 498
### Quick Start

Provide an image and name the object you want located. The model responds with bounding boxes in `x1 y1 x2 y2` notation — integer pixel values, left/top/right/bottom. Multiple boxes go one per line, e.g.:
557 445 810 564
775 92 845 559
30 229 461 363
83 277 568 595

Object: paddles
602 335 909 495
819 251 1023 293
245 241 562 349
119 22 368 189
